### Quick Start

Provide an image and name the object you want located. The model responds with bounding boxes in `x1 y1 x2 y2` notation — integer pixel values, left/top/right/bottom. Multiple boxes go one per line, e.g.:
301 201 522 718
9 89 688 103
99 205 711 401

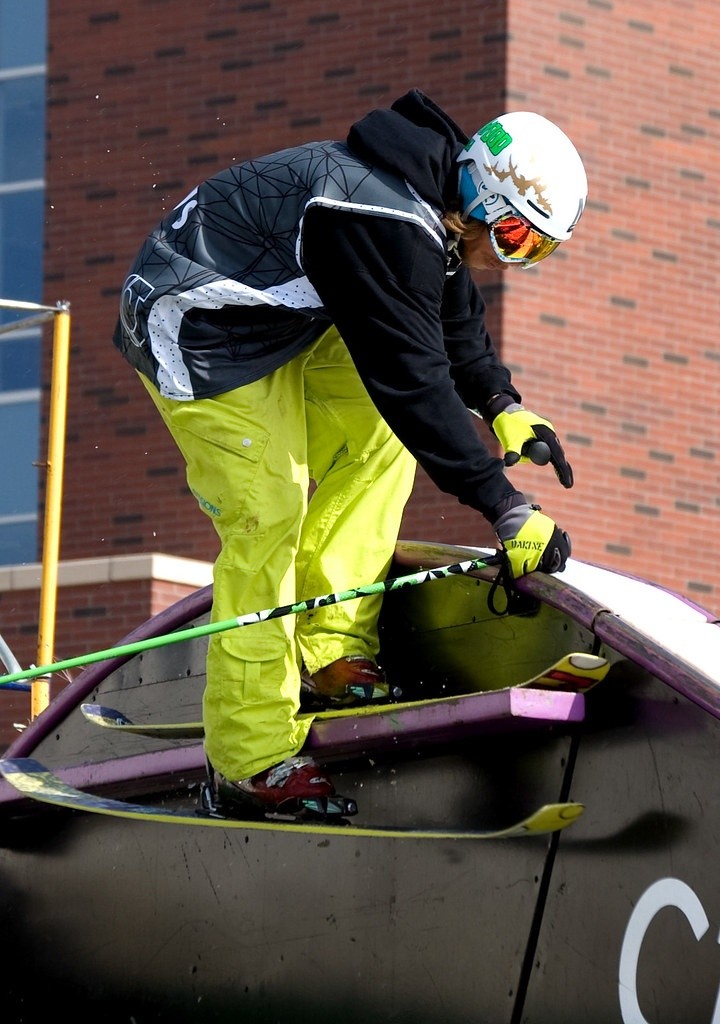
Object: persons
117 90 588 821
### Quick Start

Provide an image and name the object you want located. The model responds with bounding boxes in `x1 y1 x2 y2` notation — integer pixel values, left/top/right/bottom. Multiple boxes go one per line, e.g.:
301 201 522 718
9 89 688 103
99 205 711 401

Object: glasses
465 156 561 270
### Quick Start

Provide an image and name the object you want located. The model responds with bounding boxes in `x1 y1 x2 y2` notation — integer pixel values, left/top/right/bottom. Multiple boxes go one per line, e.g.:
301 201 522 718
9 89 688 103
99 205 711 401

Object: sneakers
299 654 403 709
201 757 357 822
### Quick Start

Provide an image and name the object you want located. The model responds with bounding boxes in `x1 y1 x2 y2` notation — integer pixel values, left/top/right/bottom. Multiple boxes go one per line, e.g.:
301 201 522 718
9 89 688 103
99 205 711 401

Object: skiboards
1 651 613 841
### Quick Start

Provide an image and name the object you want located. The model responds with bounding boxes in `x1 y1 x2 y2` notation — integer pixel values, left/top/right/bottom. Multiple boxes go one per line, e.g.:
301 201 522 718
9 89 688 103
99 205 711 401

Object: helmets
456 110 588 241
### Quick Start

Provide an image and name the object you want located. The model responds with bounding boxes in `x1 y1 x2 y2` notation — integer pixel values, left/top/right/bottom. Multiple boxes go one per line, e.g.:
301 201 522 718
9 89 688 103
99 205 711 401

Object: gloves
482 399 575 489
483 498 573 581
